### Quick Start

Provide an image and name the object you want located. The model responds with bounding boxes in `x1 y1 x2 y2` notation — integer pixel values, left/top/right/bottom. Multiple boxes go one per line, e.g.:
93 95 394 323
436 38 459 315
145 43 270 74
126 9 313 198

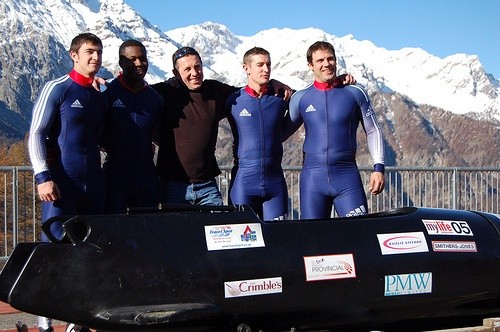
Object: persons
46 39 165 213
157 46 293 206
167 47 356 221
232 42 386 219
28 33 156 332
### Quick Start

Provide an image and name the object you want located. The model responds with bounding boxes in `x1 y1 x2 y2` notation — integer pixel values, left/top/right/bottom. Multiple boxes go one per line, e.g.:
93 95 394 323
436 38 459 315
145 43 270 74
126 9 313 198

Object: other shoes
65 324 92 332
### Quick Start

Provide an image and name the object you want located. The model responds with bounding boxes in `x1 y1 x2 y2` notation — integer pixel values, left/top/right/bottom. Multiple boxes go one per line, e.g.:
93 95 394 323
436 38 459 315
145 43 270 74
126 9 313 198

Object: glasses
173 47 196 68
